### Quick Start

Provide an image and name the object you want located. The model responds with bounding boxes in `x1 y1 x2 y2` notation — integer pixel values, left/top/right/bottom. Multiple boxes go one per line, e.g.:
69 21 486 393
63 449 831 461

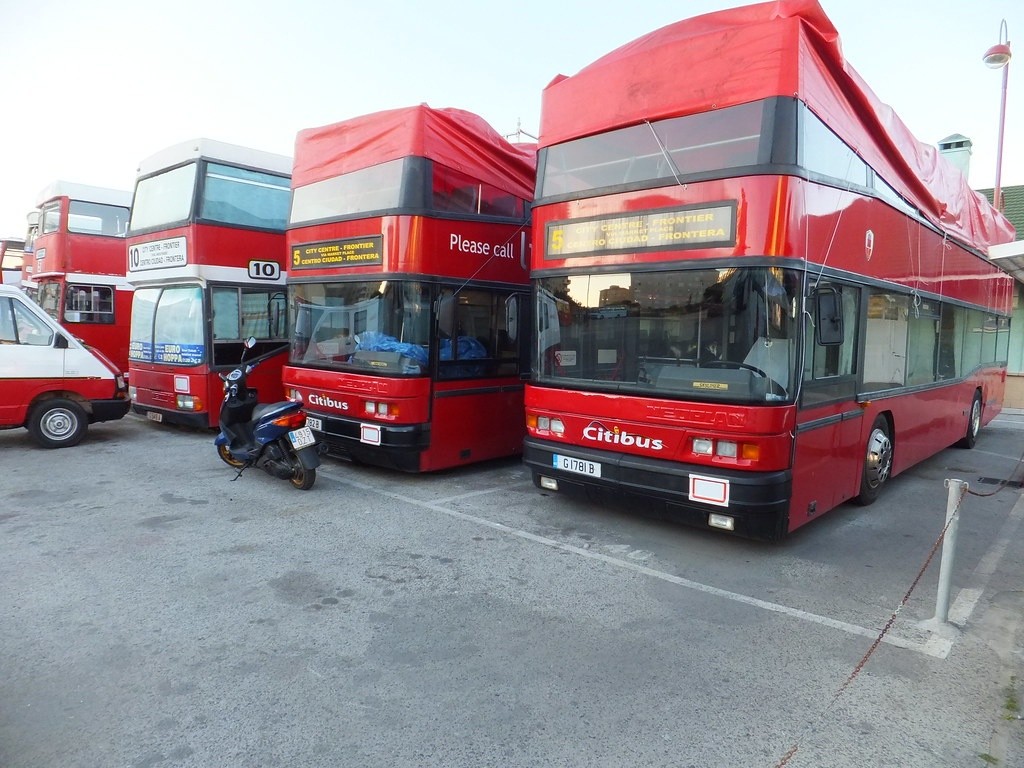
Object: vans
0 283 131 449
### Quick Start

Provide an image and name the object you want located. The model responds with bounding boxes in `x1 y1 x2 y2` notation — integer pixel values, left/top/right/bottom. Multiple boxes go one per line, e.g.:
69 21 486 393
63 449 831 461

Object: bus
126 136 328 434
0 181 133 389
279 102 624 475
521 0 1016 540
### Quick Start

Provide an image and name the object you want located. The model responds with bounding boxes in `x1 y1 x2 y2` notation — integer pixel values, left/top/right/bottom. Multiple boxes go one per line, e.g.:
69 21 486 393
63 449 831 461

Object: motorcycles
218 336 329 491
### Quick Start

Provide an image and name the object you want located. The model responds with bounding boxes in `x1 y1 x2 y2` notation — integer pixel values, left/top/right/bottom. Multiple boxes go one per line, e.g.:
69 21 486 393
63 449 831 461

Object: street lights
979 17 1012 213
